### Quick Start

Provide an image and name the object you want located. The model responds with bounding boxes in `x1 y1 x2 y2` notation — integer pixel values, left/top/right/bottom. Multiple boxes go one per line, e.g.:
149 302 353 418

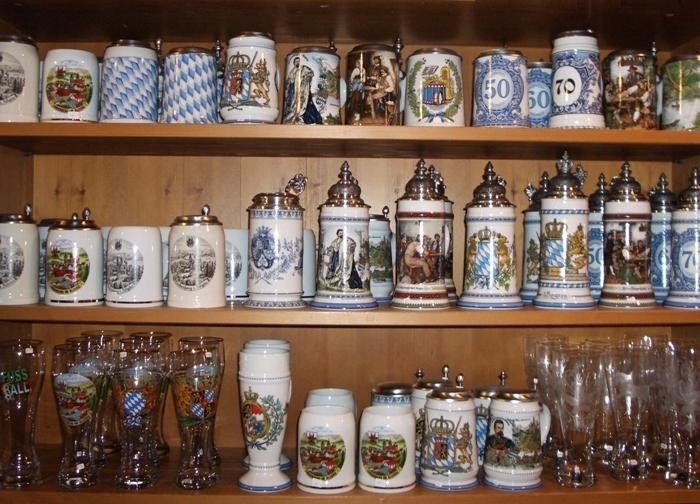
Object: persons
605 63 652 127
348 55 396 123
286 56 321 123
401 232 443 283
606 228 651 282
488 418 514 458
324 228 361 291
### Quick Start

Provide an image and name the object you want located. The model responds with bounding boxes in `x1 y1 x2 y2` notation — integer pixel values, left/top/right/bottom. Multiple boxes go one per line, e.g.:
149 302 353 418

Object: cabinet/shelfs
0 1 699 504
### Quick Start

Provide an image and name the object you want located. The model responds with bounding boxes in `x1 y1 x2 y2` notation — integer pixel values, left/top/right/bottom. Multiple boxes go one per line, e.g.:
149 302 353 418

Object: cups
3 32 221 123
304 387 354 417
485 391 544 491
357 404 419 495
0 203 249 309
52 330 225 491
295 405 356 493
235 348 293 495
402 29 605 130
421 390 480 491
1 339 48 489
521 334 700 490
242 339 292 470
